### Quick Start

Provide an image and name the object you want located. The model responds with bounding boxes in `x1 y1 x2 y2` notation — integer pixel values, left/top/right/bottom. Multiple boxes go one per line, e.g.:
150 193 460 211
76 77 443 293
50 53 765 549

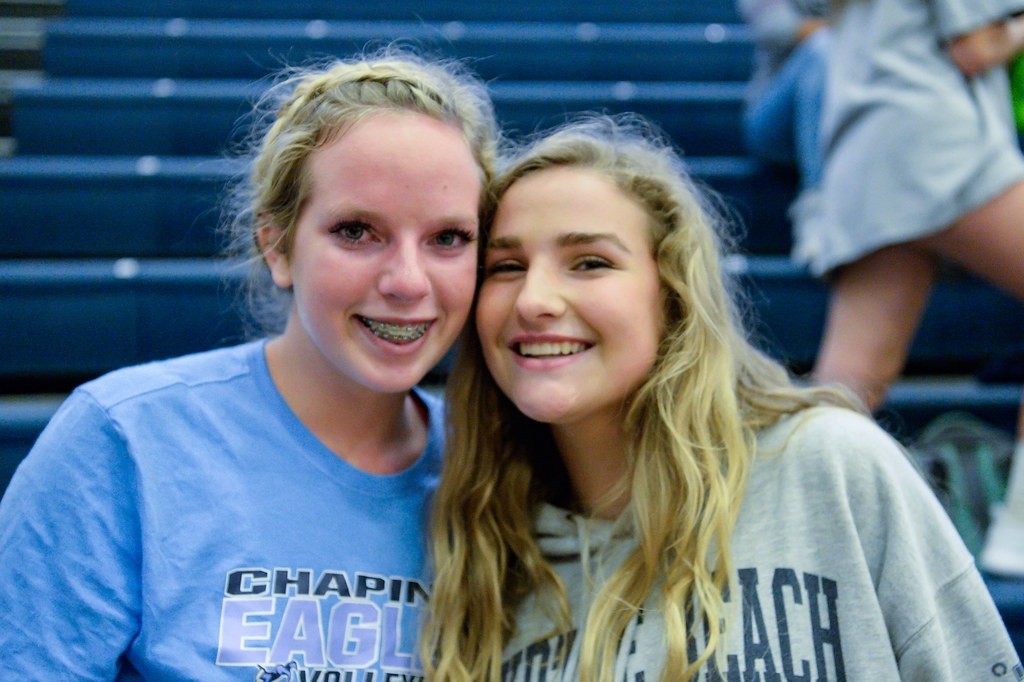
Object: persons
0 50 497 682
738 0 1024 579
416 114 1024 682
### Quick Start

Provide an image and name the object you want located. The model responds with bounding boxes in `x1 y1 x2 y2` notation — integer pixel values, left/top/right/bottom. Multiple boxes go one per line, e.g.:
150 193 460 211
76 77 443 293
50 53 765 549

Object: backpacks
905 411 1024 610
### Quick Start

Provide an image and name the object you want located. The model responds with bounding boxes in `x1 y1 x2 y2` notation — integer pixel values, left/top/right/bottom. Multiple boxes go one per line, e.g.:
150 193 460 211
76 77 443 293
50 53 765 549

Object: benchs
0 0 1024 672
